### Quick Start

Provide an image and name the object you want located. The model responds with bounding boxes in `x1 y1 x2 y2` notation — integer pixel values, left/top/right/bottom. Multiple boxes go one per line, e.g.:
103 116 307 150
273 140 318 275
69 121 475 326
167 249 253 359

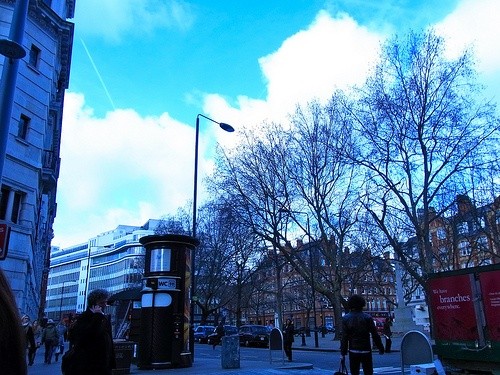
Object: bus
361 310 393 332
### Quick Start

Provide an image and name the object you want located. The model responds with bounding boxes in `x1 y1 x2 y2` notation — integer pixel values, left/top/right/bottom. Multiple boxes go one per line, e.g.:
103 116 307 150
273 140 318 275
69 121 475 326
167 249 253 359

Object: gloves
378 345 385 355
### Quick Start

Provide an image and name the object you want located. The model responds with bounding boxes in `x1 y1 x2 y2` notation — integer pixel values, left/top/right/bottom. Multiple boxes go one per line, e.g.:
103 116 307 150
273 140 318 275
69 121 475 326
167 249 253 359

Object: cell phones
92 304 97 309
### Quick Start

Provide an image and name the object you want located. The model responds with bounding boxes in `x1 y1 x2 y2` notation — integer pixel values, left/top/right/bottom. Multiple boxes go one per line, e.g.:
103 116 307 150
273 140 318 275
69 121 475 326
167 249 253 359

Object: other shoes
28 363 32 366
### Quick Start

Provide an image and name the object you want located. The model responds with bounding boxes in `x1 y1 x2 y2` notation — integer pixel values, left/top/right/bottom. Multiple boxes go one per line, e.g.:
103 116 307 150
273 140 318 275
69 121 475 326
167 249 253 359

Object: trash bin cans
373 329 382 349
222 336 240 369
112 343 136 375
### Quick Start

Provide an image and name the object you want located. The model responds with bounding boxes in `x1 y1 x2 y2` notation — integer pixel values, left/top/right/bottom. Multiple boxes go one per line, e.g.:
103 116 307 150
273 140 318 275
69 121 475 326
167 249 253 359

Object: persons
67 287 116 375
21 315 71 366
282 319 294 362
212 322 224 349
339 294 385 375
383 317 392 352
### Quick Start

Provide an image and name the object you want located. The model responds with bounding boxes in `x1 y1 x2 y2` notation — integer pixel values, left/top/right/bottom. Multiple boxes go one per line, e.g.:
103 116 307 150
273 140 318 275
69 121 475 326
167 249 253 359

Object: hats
47 319 52 323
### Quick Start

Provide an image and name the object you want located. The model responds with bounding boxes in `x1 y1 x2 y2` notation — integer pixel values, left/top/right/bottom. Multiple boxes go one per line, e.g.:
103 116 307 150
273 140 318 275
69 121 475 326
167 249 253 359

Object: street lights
59 280 76 321
278 207 319 347
189 113 235 355
83 245 111 313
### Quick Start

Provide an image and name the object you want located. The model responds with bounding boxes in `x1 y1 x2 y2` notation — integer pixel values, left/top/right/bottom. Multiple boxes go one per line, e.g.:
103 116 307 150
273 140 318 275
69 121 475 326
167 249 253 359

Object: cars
297 326 311 333
194 324 275 348
319 326 336 332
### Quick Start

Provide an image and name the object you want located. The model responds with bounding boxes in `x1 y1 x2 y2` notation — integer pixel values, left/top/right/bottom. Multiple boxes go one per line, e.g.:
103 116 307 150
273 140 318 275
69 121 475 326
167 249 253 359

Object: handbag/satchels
59 345 64 354
334 356 347 375
61 349 73 375
55 346 61 354
